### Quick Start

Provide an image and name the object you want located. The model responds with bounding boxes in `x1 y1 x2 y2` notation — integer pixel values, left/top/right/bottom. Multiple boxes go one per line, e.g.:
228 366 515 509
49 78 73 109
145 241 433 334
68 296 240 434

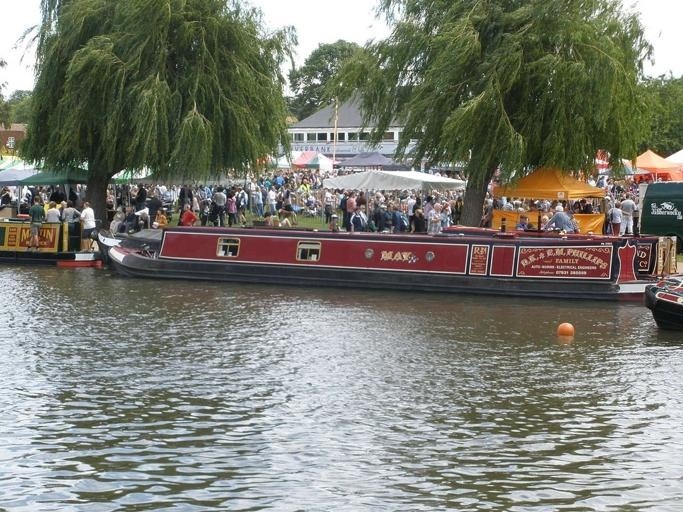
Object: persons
324 189 426 234
424 170 468 233
107 170 360 232
1 184 96 251
483 193 594 234
573 176 643 237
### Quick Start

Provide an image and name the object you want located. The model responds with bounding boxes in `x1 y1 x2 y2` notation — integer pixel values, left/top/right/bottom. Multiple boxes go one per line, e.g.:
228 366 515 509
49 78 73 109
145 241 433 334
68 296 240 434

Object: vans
639 181 683 254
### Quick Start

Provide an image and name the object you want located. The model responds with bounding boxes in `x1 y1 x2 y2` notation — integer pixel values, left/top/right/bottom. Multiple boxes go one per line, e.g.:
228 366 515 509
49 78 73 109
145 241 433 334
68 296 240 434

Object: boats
643 275 683 331
0 208 103 269
90 225 683 302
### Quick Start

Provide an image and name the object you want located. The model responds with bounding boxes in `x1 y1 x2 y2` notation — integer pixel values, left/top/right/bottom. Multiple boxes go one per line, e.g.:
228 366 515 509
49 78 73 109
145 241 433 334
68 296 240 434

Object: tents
491 162 607 235
1 170 89 212
323 171 465 218
